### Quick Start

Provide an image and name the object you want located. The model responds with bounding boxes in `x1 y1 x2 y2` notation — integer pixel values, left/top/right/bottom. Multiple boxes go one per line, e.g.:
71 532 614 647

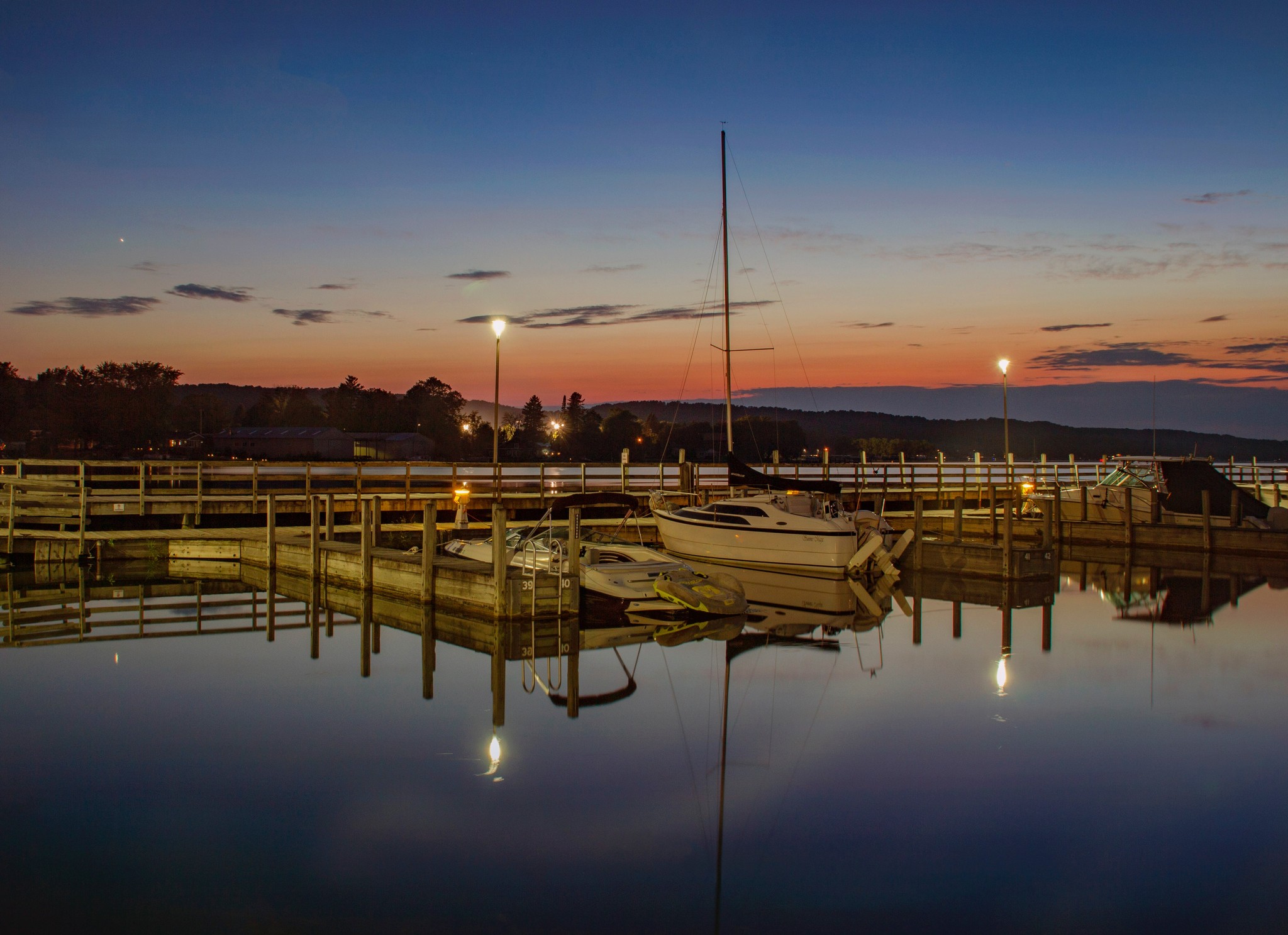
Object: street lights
491 319 506 498
997 358 1011 489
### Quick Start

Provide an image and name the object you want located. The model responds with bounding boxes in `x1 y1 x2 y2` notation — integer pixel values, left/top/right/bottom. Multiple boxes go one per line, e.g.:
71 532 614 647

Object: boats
444 492 747 615
1018 374 1288 532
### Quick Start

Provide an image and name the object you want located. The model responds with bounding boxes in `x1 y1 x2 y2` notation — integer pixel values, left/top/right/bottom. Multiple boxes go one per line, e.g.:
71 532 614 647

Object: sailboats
672 554 864 935
647 122 916 577
